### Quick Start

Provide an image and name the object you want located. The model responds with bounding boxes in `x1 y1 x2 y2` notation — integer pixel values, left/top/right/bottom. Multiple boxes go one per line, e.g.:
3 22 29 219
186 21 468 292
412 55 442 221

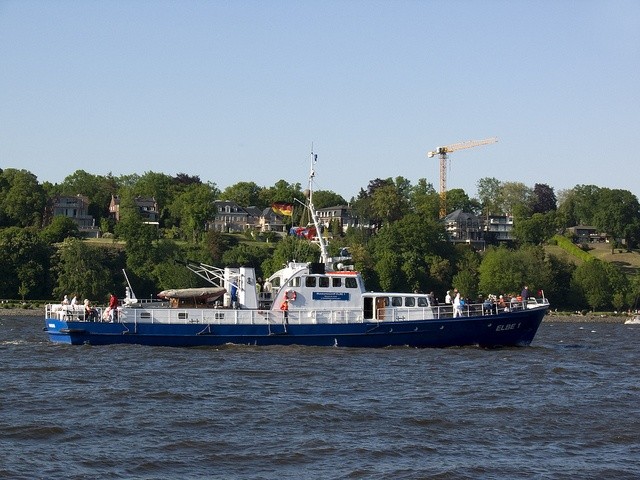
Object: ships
41 140 551 350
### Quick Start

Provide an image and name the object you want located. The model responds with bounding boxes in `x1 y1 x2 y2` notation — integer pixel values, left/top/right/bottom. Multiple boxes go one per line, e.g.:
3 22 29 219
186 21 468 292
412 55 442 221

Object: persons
453 293 462 318
484 294 522 314
521 286 530 310
257 277 266 299
460 296 464 311
71 296 78 305
431 292 438 306
61 295 69 305
109 293 117 323
263 278 272 299
83 299 98 319
445 291 451 305
280 301 288 324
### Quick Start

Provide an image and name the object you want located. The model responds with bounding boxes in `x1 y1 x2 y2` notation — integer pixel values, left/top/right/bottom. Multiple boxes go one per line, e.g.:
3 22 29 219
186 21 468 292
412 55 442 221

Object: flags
290 227 316 239
272 204 292 216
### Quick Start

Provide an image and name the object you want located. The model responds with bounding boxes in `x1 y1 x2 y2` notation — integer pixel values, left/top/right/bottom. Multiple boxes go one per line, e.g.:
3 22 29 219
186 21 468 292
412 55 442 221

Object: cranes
427 136 501 223
